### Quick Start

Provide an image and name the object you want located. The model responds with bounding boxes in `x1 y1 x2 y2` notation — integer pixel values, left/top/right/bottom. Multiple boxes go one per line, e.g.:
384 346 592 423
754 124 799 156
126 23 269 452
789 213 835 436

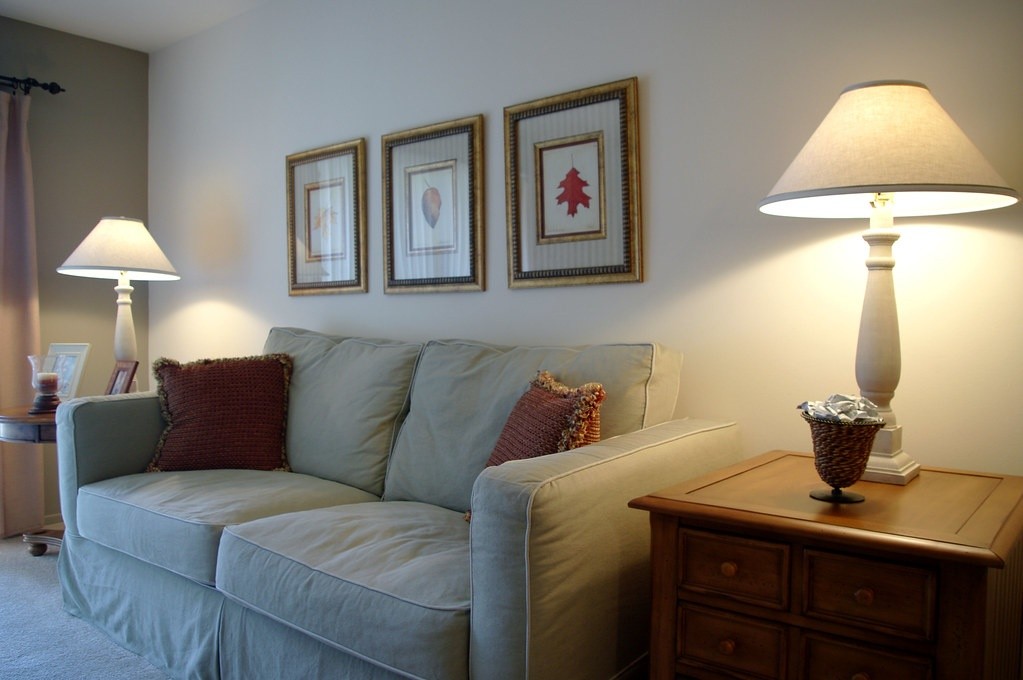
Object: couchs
54 326 696 680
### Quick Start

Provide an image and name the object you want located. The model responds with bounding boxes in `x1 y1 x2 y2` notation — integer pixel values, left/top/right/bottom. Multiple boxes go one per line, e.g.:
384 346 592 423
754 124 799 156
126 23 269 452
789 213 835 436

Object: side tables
0 405 72 557
627 449 1020 678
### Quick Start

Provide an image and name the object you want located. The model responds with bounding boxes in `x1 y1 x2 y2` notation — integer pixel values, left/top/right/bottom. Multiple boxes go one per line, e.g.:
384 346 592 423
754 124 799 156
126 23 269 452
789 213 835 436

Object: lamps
56 215 180 390
760 79 1019 485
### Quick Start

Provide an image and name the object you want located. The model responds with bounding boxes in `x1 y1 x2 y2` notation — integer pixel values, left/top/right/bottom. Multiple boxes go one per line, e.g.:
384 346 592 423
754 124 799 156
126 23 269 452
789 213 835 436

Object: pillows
463 368 609 527
146 348 295 475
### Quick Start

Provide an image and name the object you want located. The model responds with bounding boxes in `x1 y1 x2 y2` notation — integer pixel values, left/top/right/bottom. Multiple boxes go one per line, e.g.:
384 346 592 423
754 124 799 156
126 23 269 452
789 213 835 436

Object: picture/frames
286 136 369 294
382 114 487 295
106 359 140 396
40 342 91 399
502 75 644 290
